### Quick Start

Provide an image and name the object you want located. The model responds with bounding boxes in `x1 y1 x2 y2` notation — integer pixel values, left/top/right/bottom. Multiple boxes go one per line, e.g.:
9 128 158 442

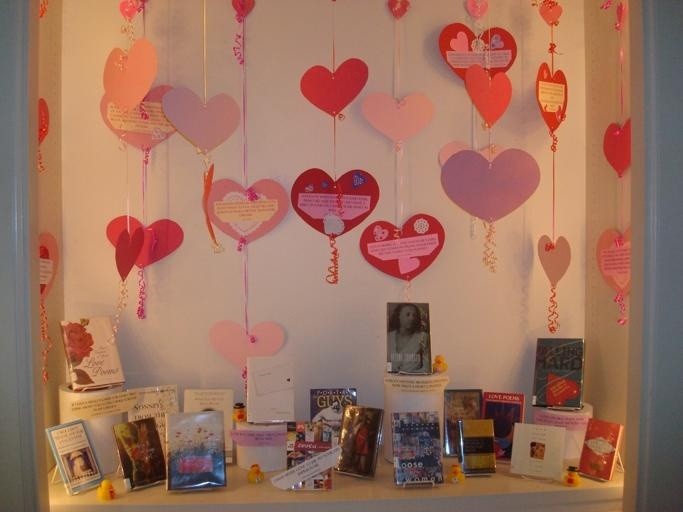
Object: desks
47 449 624 512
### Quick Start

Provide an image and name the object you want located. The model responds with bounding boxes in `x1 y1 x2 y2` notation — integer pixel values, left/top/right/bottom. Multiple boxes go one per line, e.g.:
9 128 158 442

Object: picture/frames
46 420 103 497
